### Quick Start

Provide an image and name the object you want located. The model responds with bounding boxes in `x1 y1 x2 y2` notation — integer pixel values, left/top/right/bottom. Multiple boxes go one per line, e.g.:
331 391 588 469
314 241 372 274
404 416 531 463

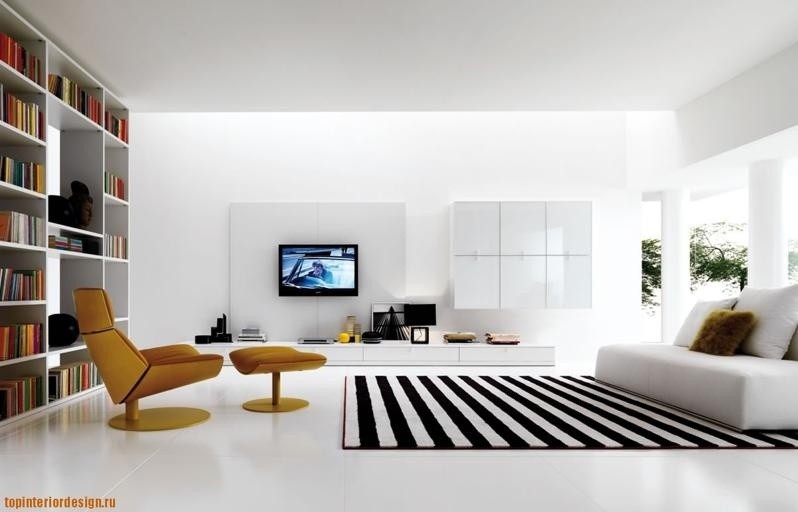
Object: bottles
354 323 361 342
346 315 355 342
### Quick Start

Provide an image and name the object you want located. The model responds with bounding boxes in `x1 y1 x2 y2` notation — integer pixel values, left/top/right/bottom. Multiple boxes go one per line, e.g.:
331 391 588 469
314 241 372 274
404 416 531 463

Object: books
1 30 129 422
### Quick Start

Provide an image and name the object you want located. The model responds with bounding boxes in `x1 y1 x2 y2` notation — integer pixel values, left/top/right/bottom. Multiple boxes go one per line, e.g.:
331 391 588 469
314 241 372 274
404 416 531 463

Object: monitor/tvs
278 243 359 297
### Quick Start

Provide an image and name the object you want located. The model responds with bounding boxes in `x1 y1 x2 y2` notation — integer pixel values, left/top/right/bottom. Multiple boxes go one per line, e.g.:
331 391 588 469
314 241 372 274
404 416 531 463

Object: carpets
342 374 798 450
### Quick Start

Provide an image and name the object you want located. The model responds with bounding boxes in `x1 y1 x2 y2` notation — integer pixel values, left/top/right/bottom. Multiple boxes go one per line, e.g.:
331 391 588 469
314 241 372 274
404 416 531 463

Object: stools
230 347 326 414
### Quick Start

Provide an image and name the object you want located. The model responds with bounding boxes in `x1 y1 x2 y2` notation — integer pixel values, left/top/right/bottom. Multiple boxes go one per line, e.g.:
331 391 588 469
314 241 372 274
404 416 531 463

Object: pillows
673 296 738 347
689 306 757 356
732 283 798 361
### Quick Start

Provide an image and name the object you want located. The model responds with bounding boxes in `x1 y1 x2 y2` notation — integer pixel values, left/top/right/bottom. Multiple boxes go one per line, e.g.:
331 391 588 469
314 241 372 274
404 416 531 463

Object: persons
308 261 332 284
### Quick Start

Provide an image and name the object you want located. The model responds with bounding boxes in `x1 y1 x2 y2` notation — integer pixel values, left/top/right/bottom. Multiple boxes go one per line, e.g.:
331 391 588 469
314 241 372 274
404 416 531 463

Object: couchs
595 302 798 433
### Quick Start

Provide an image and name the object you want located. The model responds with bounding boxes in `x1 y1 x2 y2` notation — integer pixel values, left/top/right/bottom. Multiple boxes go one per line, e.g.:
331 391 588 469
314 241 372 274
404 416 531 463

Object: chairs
72 288 224 433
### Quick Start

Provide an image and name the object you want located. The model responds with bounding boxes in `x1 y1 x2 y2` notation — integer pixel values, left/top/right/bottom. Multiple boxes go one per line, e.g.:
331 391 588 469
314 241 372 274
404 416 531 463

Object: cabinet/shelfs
0 1 129 429
189 338 557 366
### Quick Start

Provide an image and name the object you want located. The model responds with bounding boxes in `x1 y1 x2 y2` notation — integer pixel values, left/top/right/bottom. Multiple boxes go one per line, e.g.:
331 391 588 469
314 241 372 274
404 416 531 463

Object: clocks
410 327 429 344
